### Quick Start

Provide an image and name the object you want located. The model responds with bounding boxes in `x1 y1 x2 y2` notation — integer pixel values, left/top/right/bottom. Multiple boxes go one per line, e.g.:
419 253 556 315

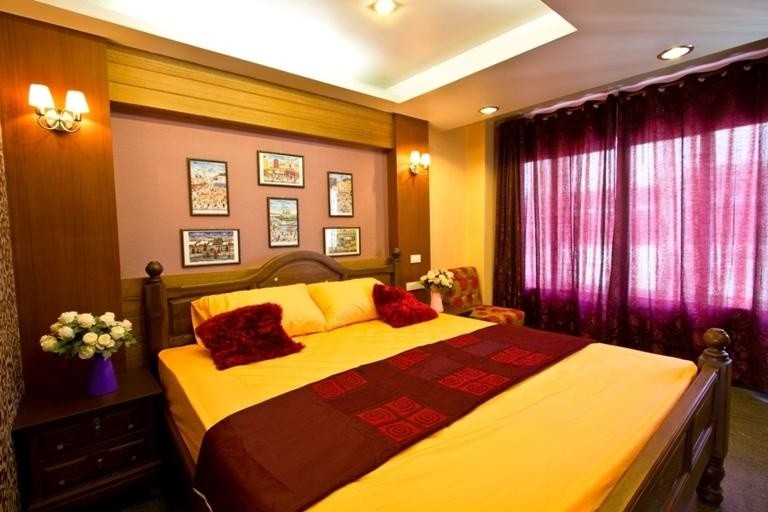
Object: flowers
420 268 455 288
39 310 137 362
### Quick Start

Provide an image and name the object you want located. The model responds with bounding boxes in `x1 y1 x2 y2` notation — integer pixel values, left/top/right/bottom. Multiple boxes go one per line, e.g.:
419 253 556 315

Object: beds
141 248 733 512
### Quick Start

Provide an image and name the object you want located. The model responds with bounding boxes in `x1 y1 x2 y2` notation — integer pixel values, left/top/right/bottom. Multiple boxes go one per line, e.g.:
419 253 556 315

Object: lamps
28 83 90 134
409 150 431 175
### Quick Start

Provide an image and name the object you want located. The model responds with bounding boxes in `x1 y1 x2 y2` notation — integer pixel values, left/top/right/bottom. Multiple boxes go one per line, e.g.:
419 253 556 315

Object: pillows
195 303 306 370
307 276 385 332
191 283 326 352
372 283 439 328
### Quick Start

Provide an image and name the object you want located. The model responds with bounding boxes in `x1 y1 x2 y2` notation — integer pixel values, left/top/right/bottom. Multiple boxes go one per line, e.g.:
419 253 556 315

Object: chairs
440 266 526 326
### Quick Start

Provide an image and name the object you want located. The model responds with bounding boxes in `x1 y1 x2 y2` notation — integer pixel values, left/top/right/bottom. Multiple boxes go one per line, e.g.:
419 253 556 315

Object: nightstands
420 299 472 317
11 362 165 512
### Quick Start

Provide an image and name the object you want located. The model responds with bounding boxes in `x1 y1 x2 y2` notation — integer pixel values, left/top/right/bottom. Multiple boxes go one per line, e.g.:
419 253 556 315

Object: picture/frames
266 197 300 248
323 226 361 257
179 228 241 268
186 158 230 217
256 150 305 188
327 171 354 218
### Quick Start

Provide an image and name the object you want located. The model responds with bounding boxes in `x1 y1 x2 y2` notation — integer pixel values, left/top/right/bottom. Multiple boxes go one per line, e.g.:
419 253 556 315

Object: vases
86 353 120 397
430 288 444 312
